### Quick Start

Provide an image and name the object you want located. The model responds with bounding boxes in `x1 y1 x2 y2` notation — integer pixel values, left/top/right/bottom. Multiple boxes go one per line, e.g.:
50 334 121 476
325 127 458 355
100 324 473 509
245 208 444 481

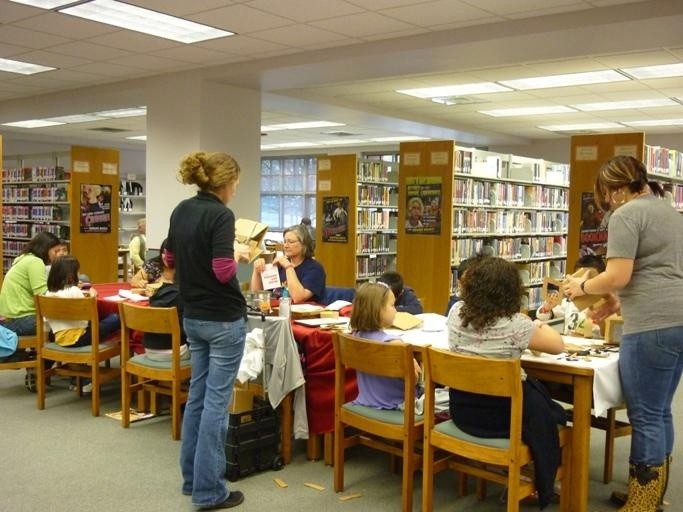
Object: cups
584 318 593 338
257 290 271 316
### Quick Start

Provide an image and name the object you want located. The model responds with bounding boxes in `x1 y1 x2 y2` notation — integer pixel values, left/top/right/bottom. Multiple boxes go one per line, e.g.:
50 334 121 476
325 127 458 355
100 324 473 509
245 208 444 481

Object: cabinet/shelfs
315 153 399 289
565 132 682 270
396 139 569 316
0 144 121 285
119 193 145 231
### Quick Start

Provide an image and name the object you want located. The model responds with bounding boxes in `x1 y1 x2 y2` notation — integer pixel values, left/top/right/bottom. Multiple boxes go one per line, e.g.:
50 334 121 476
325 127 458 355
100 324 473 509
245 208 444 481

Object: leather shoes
219 491 244 508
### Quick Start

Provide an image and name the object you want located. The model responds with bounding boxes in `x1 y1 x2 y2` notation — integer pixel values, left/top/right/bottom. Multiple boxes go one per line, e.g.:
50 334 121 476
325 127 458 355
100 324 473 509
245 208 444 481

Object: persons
348 279 428 411
563 155 683 512
378 270 424 314
129 237 177 289
300 218 316 247
1 231 62 398
41 254 121 391
81 183 111 233
535 253 622 340
324 199 347 237
445 260 479 316
446 256 574 506
144 269 191 361
580 199 613 258
248 221 326 303
406 193 439 230
164 150 245 509
51 236 92 282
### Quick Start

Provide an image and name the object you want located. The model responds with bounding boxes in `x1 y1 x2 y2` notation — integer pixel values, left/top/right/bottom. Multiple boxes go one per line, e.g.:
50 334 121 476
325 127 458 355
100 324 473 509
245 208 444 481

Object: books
355 157 398 284
643 142 683 214
257 157 317 230
448 146 569 260
516 261 569 312
0 165 70 274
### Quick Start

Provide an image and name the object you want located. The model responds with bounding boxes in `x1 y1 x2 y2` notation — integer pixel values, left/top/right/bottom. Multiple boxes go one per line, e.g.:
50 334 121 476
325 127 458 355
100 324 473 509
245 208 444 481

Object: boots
610 455 673 512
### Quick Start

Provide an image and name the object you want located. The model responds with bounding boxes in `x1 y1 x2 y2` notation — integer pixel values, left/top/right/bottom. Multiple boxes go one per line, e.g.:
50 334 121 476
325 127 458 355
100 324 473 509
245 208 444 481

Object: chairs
419 344 574 512
117 301 192 439
331 333 465 509
248 315 304 466
33 292 139 417
567 316 640 485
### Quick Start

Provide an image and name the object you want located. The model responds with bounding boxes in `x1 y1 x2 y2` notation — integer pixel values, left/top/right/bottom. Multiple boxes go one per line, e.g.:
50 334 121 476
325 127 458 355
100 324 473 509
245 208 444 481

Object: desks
308 321 633 512
71 281 409 461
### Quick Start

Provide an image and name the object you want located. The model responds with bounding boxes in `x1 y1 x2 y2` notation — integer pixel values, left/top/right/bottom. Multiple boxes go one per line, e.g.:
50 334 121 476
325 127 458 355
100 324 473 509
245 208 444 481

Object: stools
0 334 51 386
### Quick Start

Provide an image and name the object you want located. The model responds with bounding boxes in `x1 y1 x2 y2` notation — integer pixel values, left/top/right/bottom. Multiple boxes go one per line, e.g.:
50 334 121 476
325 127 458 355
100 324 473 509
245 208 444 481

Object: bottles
278 285 291 319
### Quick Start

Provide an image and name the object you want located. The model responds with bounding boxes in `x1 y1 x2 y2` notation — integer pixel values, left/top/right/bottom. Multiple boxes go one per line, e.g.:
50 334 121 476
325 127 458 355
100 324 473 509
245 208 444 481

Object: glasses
284 238 300 244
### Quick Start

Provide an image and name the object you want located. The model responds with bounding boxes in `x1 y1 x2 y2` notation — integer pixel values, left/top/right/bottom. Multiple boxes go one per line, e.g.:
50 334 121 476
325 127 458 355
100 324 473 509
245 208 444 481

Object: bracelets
285 264 293 269
